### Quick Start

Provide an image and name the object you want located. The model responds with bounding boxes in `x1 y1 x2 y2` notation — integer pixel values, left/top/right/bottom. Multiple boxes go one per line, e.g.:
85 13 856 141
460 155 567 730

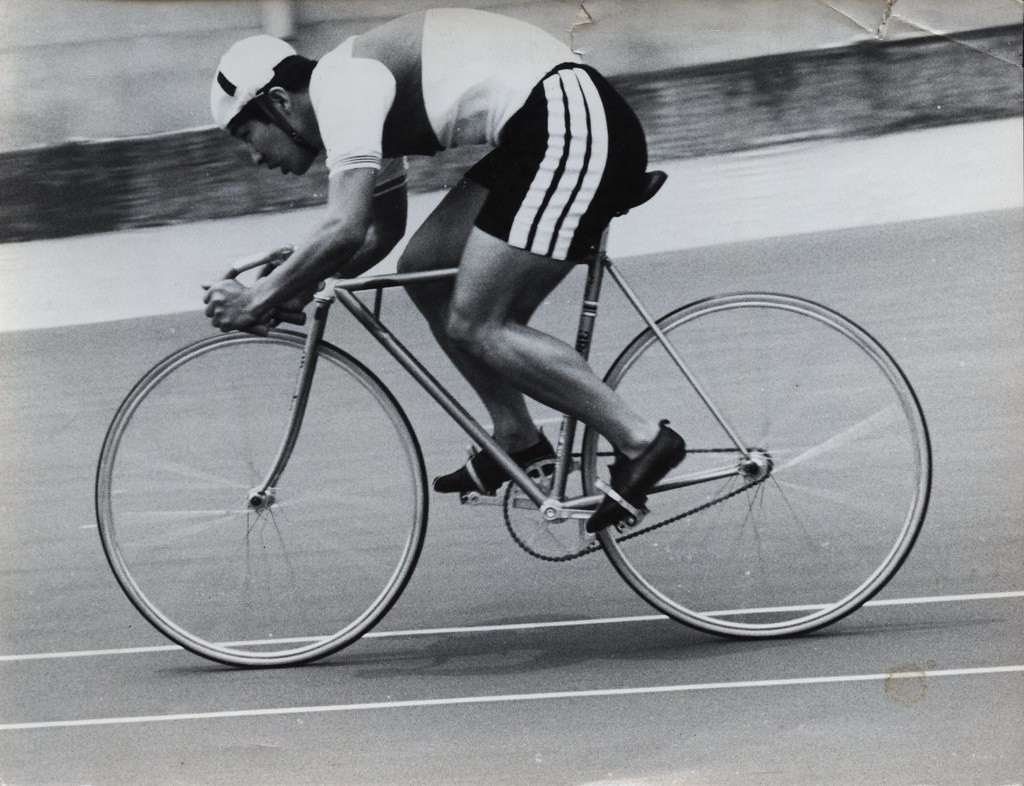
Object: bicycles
94 170 936 669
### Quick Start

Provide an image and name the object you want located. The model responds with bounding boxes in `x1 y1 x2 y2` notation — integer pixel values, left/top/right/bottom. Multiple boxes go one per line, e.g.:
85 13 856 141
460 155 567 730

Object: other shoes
433 427 559 494
586 419 688 532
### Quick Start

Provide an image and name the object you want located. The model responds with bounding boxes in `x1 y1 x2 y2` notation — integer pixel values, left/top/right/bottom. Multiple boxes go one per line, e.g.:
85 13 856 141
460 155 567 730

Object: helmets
212 34 297 131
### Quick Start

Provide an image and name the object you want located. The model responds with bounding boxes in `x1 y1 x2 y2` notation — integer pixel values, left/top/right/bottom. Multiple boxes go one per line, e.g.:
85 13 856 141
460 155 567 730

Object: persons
201 6 687 532
